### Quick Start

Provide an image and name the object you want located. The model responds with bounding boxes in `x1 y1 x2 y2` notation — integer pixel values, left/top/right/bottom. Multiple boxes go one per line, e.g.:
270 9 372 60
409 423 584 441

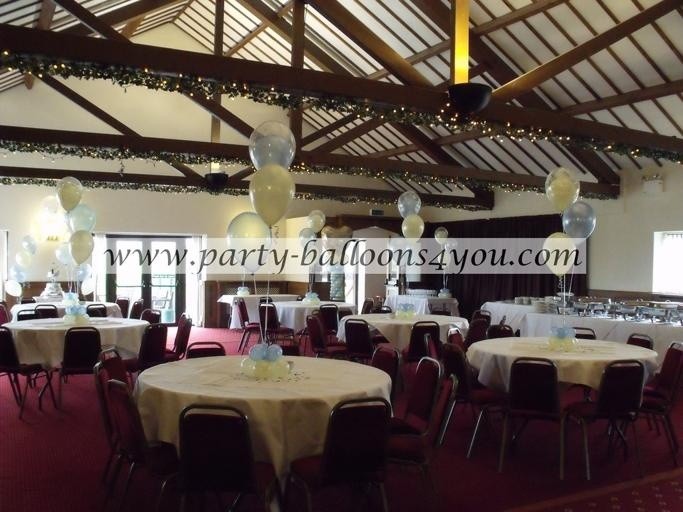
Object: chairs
0 295 683 512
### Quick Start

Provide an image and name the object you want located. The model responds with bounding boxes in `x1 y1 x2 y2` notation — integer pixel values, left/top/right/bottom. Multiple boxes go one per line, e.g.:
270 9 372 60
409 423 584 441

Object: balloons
226 121 353 378
543 167 596 351
4 176 96 323
396 191 457 319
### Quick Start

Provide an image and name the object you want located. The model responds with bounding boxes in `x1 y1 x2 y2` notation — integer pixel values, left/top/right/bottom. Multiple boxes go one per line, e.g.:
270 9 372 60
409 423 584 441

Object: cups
515 296 539 306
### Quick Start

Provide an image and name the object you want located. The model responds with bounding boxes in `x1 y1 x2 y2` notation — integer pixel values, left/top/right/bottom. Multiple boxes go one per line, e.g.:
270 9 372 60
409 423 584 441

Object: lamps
450 0 492 112
204 173 229 192
